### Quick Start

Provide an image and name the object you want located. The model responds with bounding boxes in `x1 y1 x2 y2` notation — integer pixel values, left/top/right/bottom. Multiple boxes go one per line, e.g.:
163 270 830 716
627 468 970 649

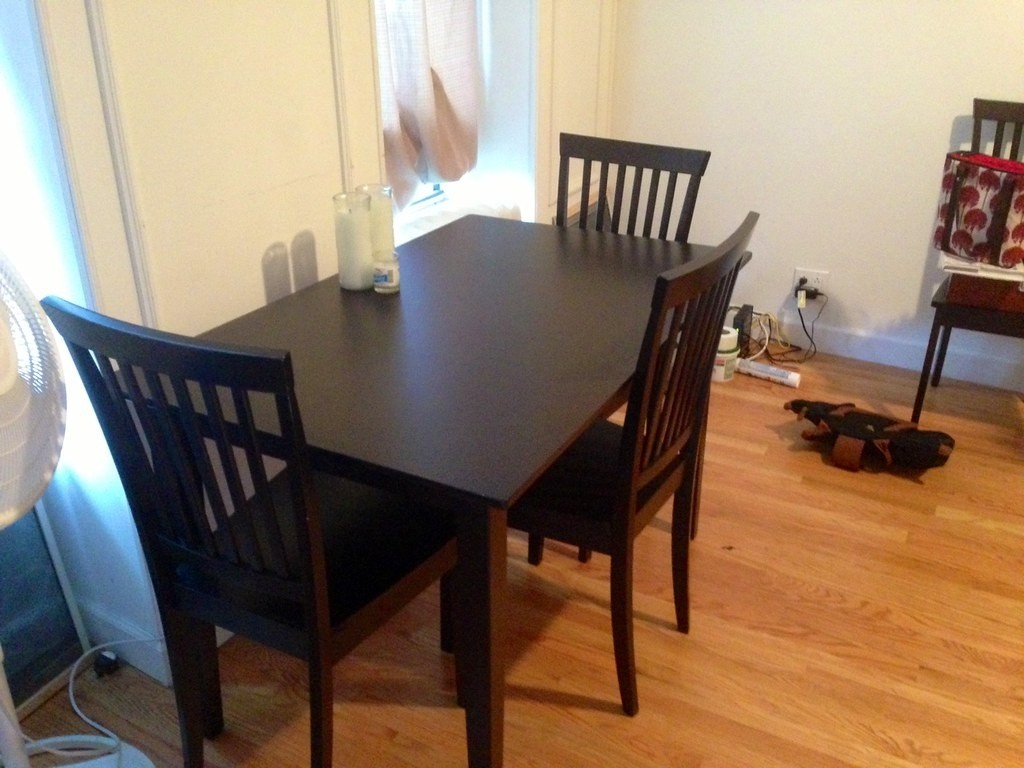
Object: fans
0 251 155 768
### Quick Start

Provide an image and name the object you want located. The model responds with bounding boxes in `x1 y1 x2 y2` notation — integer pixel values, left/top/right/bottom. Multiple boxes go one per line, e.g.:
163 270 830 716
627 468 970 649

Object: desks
115 213 753 767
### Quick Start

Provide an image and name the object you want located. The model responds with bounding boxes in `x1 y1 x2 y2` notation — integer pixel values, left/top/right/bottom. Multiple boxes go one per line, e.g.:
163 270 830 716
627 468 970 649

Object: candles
332 191 374 291
355 182 394 262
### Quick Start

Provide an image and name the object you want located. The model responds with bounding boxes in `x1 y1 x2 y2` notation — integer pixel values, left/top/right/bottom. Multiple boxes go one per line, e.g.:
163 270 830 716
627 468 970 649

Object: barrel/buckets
711 344 741 382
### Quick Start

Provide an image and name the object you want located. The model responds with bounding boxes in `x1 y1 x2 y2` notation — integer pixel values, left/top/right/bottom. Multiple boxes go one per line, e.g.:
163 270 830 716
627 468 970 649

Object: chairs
37 293 465 768
910 97 1024 424
555 133 713 564
438 211 760 717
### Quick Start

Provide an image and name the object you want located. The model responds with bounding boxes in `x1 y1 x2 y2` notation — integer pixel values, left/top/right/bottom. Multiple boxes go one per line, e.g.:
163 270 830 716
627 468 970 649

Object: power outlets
791 268 824 298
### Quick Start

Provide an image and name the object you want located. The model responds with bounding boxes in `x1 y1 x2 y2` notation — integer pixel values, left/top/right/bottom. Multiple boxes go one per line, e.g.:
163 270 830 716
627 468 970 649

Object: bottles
372 251 400 294
